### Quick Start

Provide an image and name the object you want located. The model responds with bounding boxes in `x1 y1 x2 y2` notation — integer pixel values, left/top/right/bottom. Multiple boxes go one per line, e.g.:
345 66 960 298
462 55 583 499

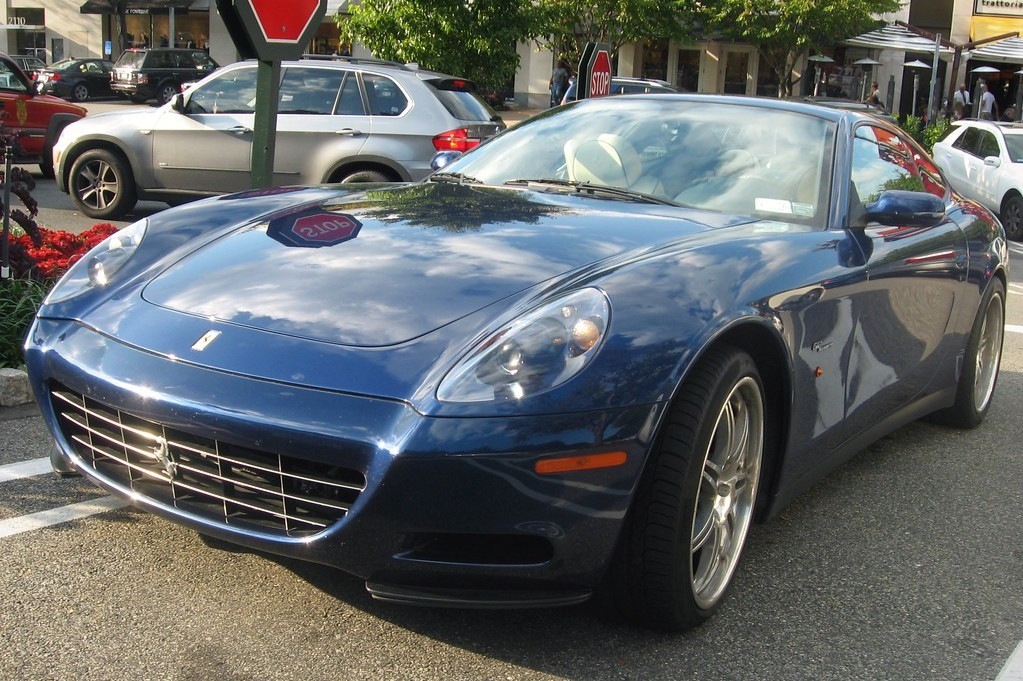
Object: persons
856 81 884 108
332 44 351 61
954 84 973 120
549 61 569 106
922 104 928 122
980 84 999 121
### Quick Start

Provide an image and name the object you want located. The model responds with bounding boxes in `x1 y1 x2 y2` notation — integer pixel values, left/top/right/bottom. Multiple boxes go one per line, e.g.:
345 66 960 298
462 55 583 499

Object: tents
838 23 1023 94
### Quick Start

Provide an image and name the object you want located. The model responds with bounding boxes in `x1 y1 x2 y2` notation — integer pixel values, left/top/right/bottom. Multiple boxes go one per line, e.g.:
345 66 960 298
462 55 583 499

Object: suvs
805 94 898 127
108 47 221 105
933 118 1023 243
560 77 688 108
52 54 506 221
0 51 88 181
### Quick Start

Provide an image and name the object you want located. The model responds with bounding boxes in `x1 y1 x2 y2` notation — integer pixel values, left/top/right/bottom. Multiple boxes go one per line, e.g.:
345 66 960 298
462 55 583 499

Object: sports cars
22 95 1009 634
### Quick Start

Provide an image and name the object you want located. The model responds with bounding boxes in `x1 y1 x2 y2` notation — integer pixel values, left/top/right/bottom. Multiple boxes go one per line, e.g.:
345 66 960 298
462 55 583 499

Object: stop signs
589 51 610 98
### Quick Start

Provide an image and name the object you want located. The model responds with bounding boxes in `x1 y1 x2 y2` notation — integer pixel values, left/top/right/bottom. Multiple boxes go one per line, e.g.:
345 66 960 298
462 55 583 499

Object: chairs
573 133 665 201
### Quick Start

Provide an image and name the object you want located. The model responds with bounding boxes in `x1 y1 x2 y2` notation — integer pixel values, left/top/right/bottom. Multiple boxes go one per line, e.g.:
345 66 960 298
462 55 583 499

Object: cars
32 57 130 103
8 54 48 80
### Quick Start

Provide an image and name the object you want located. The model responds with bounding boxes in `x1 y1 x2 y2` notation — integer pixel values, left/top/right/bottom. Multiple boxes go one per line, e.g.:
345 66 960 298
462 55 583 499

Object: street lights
902 61 933 120
807 54 836 96
851 57 884 102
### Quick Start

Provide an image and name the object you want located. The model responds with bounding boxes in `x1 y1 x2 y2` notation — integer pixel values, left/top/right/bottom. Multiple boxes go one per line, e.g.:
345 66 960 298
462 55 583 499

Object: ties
962 93 966 104
